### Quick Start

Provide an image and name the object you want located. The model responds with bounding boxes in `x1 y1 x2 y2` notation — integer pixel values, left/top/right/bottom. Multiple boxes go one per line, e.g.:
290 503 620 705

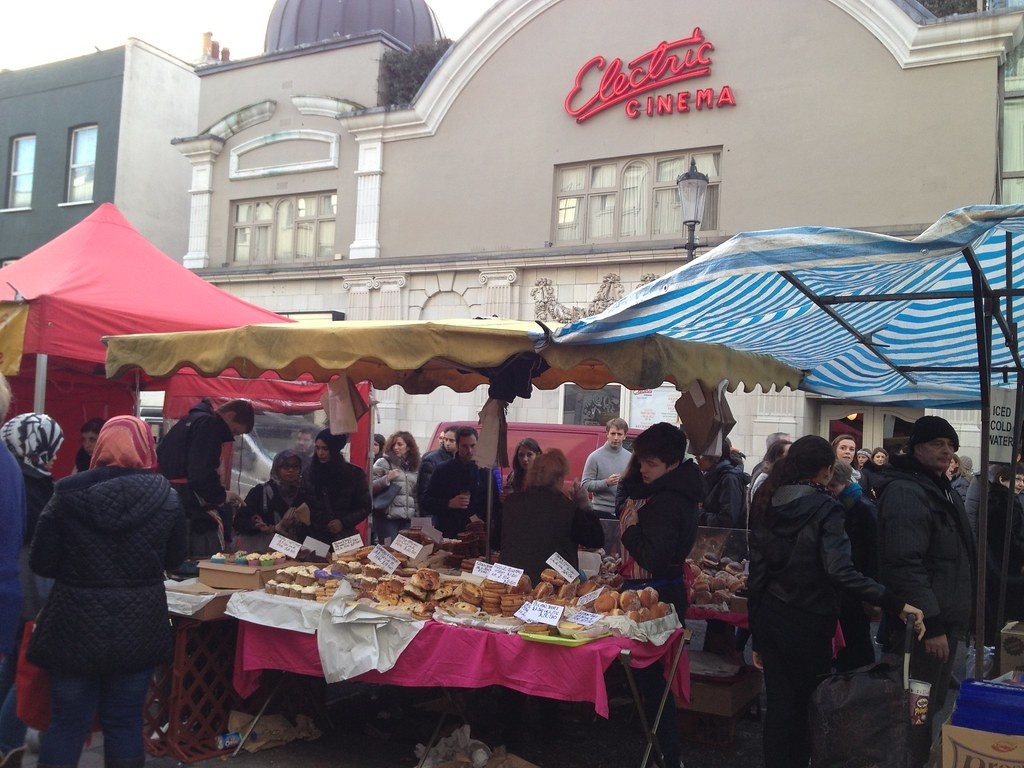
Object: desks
222 594 691 768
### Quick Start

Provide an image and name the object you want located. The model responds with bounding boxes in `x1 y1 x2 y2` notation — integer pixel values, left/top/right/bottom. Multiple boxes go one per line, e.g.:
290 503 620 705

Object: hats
960 456 972 471
315 427 347 457
909 415 958 451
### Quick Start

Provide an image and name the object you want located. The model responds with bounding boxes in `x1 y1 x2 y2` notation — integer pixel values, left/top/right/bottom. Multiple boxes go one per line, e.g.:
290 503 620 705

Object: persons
155 396 255 508
747 415 973 768
368 431 420 546
290 428 319 472
725 437 746 468
421 431 444 459
615 449 641 521
303 429 373 534
610 420 710 768
232 449 331 541
498 437 543 503
490 447 605 742
417 426 462 516
581 418 634 556
694 434 747 561
372 433 386 463
965 455 1024 650
422 424 483 539
0 371 191 768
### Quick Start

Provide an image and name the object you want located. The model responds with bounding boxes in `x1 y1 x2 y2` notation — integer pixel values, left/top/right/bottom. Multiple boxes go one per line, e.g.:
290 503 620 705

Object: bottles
215 730 257 750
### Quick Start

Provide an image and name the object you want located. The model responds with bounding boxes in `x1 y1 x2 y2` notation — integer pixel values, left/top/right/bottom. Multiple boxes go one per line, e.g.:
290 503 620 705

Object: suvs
139 406 321 502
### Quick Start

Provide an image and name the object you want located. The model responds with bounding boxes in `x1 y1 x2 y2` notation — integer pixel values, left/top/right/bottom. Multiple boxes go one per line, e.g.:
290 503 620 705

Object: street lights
676 153 711 262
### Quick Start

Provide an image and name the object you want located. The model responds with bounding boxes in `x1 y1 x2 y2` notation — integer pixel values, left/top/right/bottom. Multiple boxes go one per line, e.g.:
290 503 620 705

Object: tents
0 201 300 418
101 319 811 566
526 202 1024 681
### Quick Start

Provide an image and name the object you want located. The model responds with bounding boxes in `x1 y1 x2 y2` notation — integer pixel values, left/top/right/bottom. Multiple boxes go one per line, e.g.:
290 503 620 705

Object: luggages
808 613 929 768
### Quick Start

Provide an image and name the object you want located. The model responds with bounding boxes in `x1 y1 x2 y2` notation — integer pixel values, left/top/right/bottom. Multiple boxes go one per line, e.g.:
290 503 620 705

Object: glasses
281 463 300 470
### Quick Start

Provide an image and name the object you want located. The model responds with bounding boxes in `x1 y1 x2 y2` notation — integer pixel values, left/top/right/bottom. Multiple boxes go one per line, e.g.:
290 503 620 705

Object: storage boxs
999 621 1024 677
197 558 298 590
940 710 1023 768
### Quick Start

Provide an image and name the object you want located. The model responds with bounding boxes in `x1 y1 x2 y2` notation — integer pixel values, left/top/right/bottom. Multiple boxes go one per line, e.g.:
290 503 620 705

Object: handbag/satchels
16 618 104 735
373 456 402 511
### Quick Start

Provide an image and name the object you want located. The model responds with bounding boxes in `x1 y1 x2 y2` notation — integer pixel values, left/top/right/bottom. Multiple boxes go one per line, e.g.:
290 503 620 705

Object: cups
910 679 931 723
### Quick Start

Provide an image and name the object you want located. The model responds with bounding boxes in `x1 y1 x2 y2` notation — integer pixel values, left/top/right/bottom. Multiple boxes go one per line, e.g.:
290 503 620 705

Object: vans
426 422 646 503
